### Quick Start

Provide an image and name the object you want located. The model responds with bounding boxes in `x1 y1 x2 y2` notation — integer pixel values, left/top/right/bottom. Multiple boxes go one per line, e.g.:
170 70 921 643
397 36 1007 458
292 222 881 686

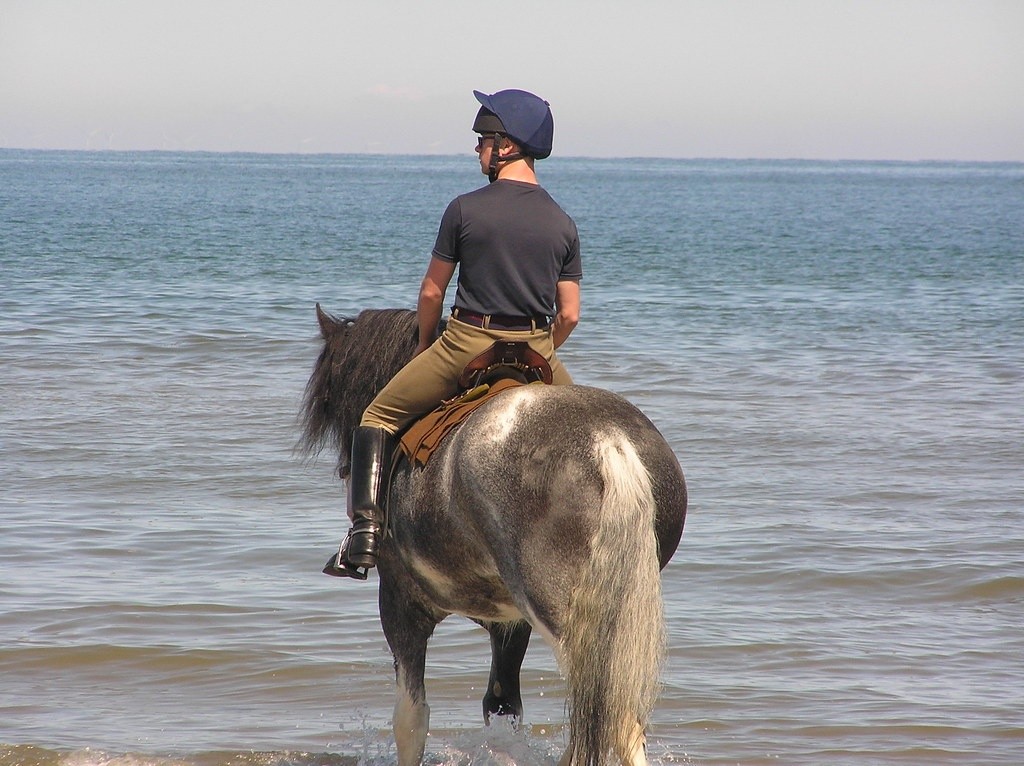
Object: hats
473 87 554 150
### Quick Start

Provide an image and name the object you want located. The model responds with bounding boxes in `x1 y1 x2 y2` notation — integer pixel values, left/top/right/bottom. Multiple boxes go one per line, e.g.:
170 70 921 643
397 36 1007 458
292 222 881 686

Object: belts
452 309 550 331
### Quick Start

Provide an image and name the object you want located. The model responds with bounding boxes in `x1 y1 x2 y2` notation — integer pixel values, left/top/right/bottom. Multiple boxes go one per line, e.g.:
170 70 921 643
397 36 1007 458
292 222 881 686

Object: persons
323 87 583 581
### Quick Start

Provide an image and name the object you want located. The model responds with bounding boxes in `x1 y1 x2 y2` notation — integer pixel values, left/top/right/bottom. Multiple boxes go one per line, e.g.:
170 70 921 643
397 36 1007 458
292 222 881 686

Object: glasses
477 137 495 147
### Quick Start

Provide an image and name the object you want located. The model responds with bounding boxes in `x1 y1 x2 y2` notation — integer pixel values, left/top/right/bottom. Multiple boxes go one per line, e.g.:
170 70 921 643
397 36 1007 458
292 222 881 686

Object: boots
322 426 393 576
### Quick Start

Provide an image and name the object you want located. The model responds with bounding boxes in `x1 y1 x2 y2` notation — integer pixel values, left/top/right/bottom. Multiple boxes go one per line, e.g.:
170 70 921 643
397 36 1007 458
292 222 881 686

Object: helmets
472 89 552 160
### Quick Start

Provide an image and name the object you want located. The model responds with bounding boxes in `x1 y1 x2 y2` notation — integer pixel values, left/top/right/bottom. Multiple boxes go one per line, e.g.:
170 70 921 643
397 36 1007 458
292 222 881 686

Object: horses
293 300 688 766
